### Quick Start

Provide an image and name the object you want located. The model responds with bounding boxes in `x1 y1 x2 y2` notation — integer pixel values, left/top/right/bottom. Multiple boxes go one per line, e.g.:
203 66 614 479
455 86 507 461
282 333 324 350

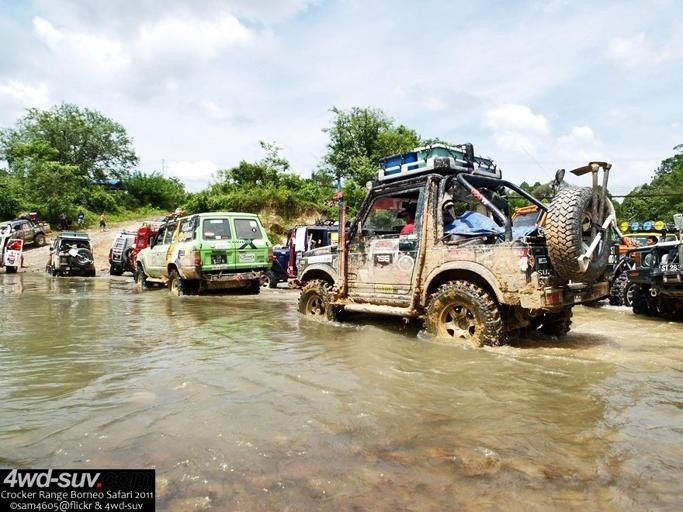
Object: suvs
133 211 274 298
45 231 96 276
108 220 166 276
0 212 52 273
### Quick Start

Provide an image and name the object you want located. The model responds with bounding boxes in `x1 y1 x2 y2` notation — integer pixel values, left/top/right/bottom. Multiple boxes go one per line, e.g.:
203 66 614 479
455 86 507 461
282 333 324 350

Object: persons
8 236 26 268
400 203 417 234
647 236 658 245
100 213 107 232
77 210 85 229
58 211 69 231
